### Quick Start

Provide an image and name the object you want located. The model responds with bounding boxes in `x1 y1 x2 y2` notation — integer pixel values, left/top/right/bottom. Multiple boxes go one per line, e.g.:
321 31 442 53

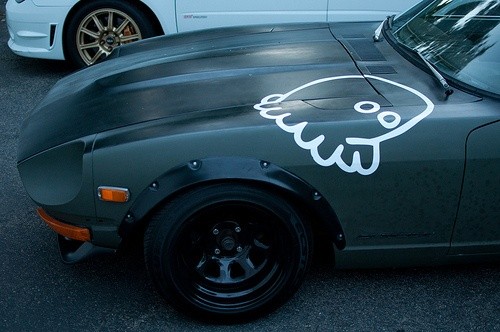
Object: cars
14 0 500 322
5 0 500 74
